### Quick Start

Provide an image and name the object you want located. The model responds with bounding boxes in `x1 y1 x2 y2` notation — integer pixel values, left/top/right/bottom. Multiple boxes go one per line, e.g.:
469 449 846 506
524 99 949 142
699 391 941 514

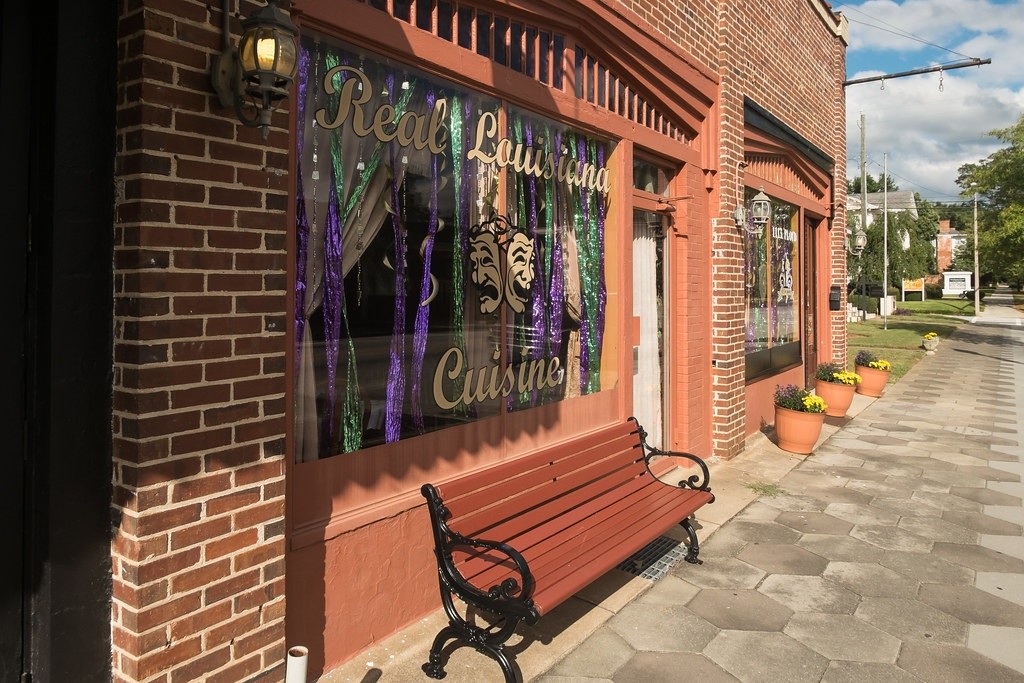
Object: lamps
733 184 771 239
844 227 868 255
211 0 300 142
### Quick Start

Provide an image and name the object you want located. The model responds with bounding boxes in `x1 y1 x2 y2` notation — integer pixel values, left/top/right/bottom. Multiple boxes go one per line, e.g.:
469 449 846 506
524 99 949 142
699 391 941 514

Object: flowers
772 383 828 413
855 350 891 371
925 332 937 340
815 362 863 386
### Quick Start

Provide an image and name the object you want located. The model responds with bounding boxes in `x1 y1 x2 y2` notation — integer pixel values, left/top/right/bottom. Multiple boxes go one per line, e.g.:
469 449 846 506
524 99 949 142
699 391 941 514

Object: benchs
420 417 715 683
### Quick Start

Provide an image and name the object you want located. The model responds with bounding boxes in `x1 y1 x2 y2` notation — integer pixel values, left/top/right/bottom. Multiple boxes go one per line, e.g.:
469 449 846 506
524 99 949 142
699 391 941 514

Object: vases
773 402 827 454
813 376 856 417
855 363 890 398
922 337 940 351
